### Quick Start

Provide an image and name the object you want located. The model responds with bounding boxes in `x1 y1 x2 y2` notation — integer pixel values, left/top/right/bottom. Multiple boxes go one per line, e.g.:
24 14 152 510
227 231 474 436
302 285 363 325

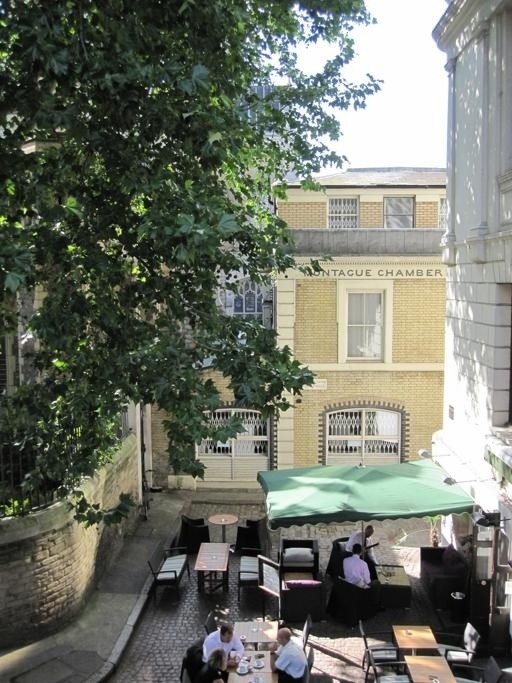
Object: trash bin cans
448 591 470 624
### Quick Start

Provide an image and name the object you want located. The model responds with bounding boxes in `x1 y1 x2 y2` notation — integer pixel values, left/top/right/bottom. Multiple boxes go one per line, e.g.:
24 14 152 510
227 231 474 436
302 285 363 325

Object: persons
197 624 244 683
342 524 379 589
270 627 307 683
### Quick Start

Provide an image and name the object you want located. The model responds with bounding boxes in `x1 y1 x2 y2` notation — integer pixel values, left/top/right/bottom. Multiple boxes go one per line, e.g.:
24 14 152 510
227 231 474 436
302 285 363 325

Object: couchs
418 546 469 612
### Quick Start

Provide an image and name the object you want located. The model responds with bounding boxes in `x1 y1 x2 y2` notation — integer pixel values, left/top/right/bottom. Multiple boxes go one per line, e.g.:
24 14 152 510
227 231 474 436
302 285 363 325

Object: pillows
442 541 465 577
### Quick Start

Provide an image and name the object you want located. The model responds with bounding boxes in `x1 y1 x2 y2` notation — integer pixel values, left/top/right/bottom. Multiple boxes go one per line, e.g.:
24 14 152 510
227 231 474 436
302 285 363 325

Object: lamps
441 472 497 485
417 448 450 460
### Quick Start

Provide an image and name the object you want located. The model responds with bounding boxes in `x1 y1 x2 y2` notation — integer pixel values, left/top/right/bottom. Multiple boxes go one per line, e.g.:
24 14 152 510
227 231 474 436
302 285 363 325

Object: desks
194 542 231 592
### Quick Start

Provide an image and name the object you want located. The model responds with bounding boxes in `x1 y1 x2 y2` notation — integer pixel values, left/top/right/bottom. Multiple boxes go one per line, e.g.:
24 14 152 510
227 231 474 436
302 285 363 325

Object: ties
364 537 367 546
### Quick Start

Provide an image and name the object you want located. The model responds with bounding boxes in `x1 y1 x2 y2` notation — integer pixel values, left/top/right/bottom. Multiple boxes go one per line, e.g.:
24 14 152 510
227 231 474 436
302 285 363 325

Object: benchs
238 548 269 604
147 540 190 603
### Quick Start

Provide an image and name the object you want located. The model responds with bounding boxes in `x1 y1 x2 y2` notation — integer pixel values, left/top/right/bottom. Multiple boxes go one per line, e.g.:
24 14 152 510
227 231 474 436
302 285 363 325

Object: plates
236 667 249 674
253 662 264 668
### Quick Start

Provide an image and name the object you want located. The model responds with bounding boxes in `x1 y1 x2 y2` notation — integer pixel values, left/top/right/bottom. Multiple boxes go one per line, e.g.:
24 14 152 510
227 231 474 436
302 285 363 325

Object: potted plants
137 494 154 521
424 514 441 547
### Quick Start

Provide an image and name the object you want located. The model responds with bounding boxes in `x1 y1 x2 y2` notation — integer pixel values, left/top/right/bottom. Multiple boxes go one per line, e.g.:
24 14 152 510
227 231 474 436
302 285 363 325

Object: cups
255 659 261 666
239 665 246 672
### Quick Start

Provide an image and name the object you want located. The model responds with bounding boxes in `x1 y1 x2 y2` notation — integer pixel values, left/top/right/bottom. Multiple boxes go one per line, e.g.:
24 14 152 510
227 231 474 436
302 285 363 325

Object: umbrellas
257 459 473 559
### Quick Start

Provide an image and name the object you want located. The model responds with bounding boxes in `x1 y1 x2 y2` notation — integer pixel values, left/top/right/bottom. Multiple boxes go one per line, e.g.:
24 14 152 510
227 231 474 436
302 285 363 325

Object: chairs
282 536 376 622
204 610 218 635
302 614 314 683
357 618 503 683
180 657 193 683
178 514 267 553
